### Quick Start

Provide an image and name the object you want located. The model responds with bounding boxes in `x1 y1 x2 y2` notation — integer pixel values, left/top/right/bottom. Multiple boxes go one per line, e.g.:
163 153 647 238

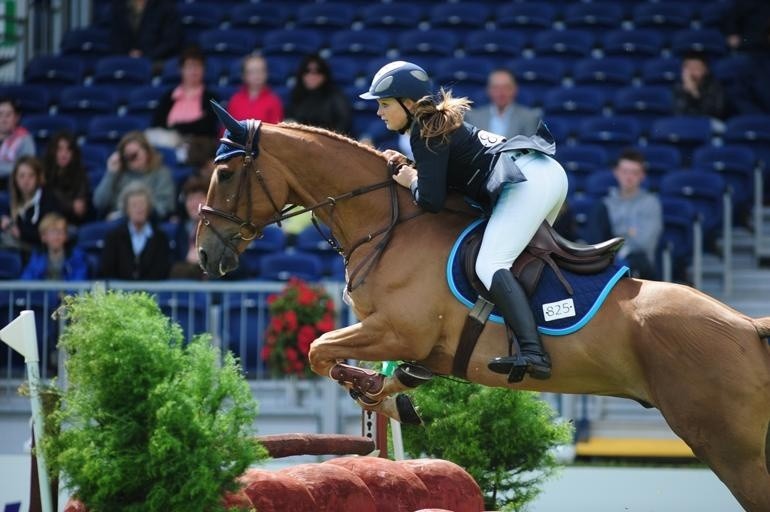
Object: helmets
356 60 434 104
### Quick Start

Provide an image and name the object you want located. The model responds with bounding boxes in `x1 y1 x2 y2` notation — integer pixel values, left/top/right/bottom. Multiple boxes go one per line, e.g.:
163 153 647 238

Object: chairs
0 1 768 380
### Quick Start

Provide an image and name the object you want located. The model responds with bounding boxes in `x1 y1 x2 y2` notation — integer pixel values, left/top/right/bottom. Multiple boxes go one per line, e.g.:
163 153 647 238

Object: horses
195 95 770 512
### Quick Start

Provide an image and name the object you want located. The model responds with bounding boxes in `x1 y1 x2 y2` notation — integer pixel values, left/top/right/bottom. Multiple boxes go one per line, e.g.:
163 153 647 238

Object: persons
720 1 770 53
111 1 171 59
676 55 729 121
604 149 665 280
356 59 569 380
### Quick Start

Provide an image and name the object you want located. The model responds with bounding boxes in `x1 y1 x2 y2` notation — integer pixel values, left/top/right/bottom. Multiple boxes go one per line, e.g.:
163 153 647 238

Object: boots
486 263 555 382
